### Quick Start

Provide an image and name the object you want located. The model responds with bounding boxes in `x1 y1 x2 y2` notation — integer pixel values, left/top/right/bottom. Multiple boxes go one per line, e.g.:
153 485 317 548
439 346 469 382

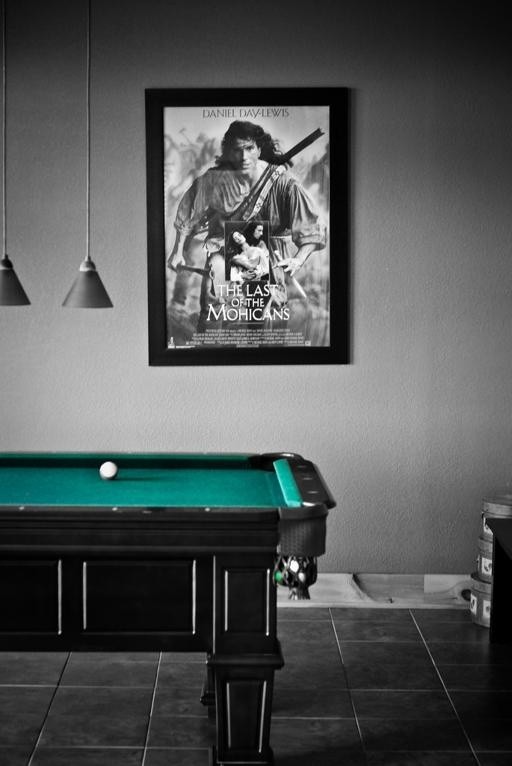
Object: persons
228 222 269 280
169 121 325 347
230 228 268 281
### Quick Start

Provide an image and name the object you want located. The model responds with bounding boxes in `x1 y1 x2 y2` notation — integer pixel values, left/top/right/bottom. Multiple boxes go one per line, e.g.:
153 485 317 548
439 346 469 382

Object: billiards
275 555 317 588
98 461 118 478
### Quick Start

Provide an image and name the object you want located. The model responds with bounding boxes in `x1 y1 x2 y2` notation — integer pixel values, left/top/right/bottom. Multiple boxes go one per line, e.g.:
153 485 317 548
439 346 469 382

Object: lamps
0 0 116 310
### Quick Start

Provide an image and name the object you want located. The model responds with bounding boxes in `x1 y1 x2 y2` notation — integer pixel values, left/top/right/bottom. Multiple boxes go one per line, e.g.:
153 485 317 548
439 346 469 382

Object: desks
0 451 339 765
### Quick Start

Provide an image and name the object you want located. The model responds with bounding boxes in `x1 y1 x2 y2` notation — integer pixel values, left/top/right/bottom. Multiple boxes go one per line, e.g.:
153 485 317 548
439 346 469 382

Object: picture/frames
142 84 353 369
